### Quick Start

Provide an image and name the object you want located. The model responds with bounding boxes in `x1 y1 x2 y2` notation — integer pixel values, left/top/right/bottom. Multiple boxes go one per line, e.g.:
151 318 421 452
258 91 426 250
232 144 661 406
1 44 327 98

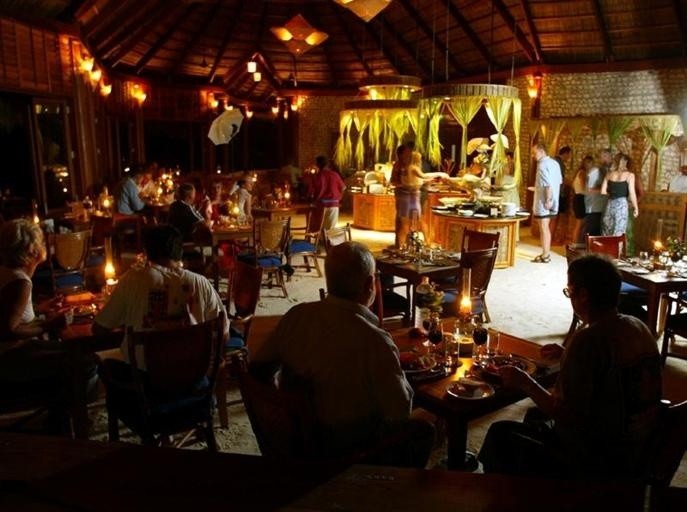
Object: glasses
563 288 579 298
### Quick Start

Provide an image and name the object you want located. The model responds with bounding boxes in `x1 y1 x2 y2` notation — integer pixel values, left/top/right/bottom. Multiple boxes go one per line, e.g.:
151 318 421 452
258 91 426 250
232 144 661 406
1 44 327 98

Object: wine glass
371 236 458 270
611 249 687 278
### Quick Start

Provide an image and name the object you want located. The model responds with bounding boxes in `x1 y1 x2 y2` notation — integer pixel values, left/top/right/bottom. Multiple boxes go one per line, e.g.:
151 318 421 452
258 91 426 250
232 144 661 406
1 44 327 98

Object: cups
212 186 291 229
151 166 182 199
51 278 119 319
69 184 115 218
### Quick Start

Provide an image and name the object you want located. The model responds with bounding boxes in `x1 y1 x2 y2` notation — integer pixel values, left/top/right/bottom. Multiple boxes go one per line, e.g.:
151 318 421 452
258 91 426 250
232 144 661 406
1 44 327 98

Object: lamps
331 0 522 178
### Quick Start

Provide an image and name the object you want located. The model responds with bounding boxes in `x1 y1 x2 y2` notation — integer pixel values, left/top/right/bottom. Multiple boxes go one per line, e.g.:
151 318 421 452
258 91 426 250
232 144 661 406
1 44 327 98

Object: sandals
530 254 551 264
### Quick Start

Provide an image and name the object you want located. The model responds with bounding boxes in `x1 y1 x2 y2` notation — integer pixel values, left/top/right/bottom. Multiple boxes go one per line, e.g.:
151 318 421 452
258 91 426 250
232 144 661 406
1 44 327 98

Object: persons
530 144 638 260
250 242 439 469
2 159 254 447
480 255 664 478
389 142 513 249
279 154 346 231
669 165 686 191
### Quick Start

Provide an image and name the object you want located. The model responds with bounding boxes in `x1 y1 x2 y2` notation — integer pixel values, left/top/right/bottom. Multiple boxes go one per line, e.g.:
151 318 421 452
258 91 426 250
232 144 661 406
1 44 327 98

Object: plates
428 193 530 219
393 275 539 401
